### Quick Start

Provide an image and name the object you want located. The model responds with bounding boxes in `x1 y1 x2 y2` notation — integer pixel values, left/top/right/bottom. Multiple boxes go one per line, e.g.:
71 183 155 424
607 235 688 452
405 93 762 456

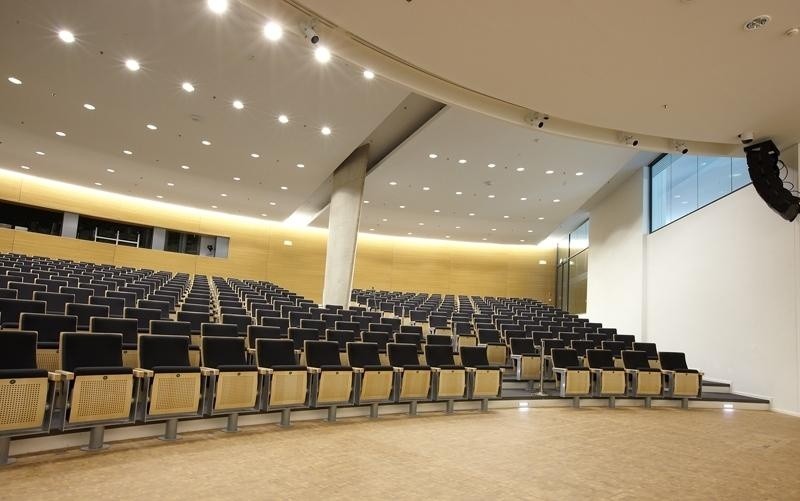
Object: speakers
744 139 800 222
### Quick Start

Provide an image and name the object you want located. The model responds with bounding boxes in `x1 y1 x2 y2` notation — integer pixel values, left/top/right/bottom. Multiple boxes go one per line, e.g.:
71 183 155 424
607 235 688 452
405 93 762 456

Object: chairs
1 251 503 460
499 290 702 411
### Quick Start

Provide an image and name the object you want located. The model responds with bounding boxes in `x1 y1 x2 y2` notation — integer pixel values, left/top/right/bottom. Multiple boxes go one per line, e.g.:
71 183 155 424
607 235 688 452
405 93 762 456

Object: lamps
672 138 690 154
523 107 550 130
616 130 640 148
298 20 320 46
